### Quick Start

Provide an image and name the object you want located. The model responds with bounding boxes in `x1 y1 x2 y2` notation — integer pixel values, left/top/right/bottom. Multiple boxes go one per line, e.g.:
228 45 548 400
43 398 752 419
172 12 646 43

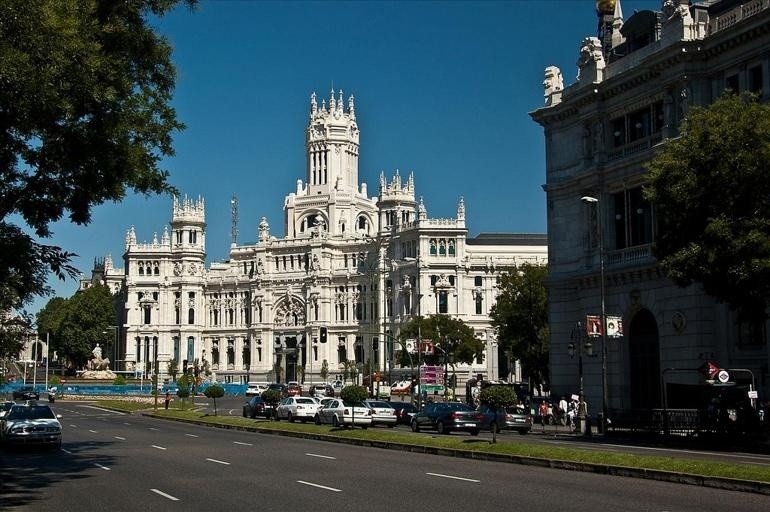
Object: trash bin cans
597 412 604 432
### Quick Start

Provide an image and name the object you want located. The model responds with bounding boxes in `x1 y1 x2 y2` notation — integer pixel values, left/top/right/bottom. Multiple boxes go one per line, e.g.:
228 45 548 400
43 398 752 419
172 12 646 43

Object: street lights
582 195 608 439
404 255 421 410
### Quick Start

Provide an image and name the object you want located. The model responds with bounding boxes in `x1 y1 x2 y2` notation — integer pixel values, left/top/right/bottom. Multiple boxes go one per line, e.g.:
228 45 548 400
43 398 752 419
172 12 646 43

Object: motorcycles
48 391 55 404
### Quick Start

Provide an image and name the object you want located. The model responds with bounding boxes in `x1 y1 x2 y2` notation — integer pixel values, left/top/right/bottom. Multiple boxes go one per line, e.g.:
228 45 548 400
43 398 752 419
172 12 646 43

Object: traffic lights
320 326 327 343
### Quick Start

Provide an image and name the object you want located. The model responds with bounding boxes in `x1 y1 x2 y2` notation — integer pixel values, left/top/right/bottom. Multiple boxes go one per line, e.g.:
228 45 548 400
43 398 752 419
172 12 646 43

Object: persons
517 400 525 408
165 388 171 410
536 395 587 432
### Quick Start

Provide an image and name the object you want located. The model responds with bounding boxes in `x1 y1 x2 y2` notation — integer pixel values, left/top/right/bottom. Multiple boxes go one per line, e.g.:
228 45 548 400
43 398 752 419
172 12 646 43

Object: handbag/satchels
560 409 565 414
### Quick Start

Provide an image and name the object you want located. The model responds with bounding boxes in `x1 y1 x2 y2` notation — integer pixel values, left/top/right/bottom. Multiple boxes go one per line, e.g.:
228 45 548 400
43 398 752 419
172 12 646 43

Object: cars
243 382 533 436
0 403 62 457
0 398 16 425
12 387 40 402
